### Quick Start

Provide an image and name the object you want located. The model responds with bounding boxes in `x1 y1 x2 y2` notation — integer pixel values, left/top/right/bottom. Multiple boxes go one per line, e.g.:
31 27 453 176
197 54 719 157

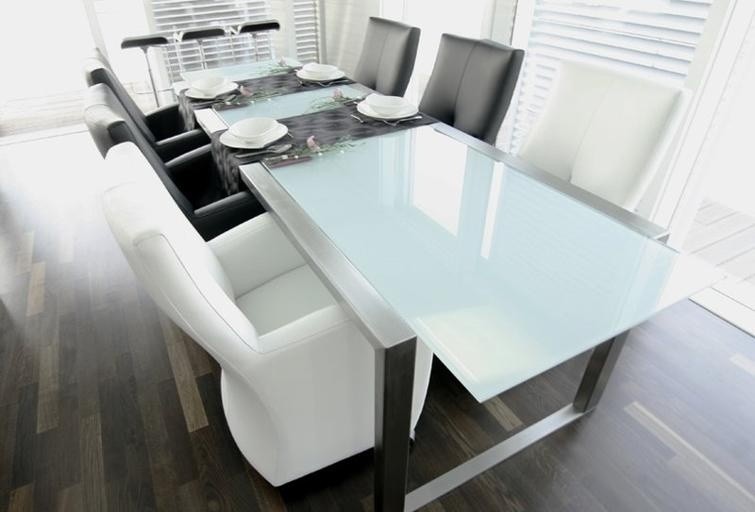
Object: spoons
193 94 236 108
235 142 292 161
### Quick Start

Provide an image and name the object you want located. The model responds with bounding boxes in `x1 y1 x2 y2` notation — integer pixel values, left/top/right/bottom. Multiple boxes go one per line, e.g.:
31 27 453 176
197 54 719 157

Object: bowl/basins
191 75 223 93
228 116 278 142
365 96 406 114
303 63 339 77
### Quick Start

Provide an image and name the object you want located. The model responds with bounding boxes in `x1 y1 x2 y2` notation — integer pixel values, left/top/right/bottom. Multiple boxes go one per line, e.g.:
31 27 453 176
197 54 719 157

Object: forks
316 80 351 88
349 114 377 125
382 116 425 128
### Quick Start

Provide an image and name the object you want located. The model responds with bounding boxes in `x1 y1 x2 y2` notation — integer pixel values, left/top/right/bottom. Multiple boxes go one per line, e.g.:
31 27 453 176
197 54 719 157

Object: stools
119 35 170 118
229 20 283 68
173 24 226 77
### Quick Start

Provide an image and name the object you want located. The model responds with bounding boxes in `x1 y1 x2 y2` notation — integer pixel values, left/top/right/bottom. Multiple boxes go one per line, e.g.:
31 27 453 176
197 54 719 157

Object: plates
356 100 418 121
184 82 238 99
296 70 344 82
219 122 289 150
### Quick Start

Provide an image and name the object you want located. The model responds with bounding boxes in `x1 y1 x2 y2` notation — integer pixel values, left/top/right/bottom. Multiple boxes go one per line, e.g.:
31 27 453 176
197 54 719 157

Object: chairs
80 83 268 246
84 46 211 166
351 15 420 100
95 138 437 490
511 56 695 217
419 32 524 147
